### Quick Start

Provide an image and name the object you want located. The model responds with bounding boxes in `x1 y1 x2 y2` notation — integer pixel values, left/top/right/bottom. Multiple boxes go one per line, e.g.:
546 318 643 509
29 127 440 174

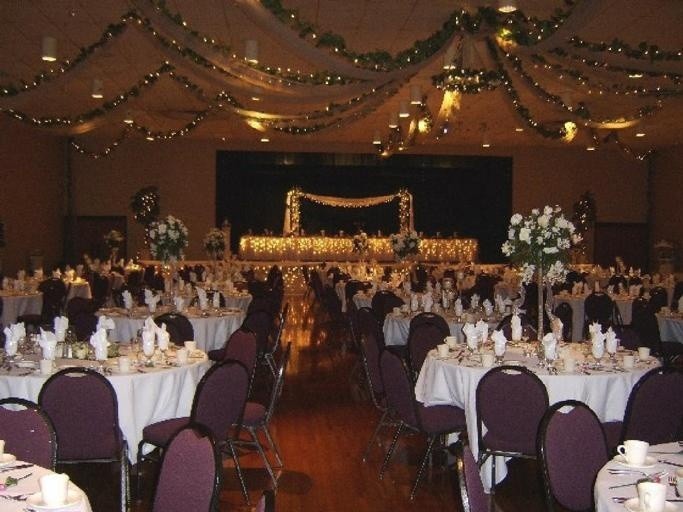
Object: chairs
381 300 404 314
359 330 436 467
72 312 109 342
347 306 382 391
321 287 346 347
240 350 286 486
240 263 286 326
137 359 251 504
536 400 613 512
606 326 642 350
410 312 448 334
496 313 533 328
376 348 465 501
0 397 55 471
151 422 218 512
620 366 683 449
303 258 683 304
583 292 614 340
408 323 441 375
41 277 68 314
371 290 395 308
248 302 287 402
472 366 550 511
66 277 109 321
38 367 132 511
122 263 204 303
16 311 50 328
219 326 258 369
499 323 539 342
154 314 191 348
554 302 573 340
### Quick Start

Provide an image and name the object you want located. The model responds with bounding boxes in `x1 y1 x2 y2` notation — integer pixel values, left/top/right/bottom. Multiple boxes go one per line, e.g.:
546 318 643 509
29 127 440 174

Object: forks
668 475 680 497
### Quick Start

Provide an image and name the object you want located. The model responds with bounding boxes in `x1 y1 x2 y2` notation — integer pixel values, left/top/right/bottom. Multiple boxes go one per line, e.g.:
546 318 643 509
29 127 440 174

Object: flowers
391 231 423 262
499 205 581 351
201 227 229 274
104 228 121 264
351 233 370 259
145 216 190 272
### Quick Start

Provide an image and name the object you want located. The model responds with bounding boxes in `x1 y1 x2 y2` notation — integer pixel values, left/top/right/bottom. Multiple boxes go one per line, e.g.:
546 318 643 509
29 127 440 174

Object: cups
481 355 494 369
623 356 634 372
95 350 108 375
495 344 505 365
617 440 649 465
591 346 603 370
563 358 575 373
606 344 617 360
638 347 649 362
117 356 130 374
143 344 155 366
637 482 667 512
437 344 448 357
410 303 418 316
176 350 188 365
159 344 168 363
545 351 555 374
38 473 71 505
39 360 52 376
455 310 462 323
485 308 492 321
125 301 133 317
467 339 477 355
148 301 156 317
184 341 197 355
5 342 16 361
444 337 456 348
393 307 401 318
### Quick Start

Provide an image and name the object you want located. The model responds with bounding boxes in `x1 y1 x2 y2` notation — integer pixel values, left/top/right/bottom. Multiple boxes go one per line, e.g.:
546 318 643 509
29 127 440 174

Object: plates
25 490 80 511
624 499 676 512
613 454 658 469
15 361 35 367
